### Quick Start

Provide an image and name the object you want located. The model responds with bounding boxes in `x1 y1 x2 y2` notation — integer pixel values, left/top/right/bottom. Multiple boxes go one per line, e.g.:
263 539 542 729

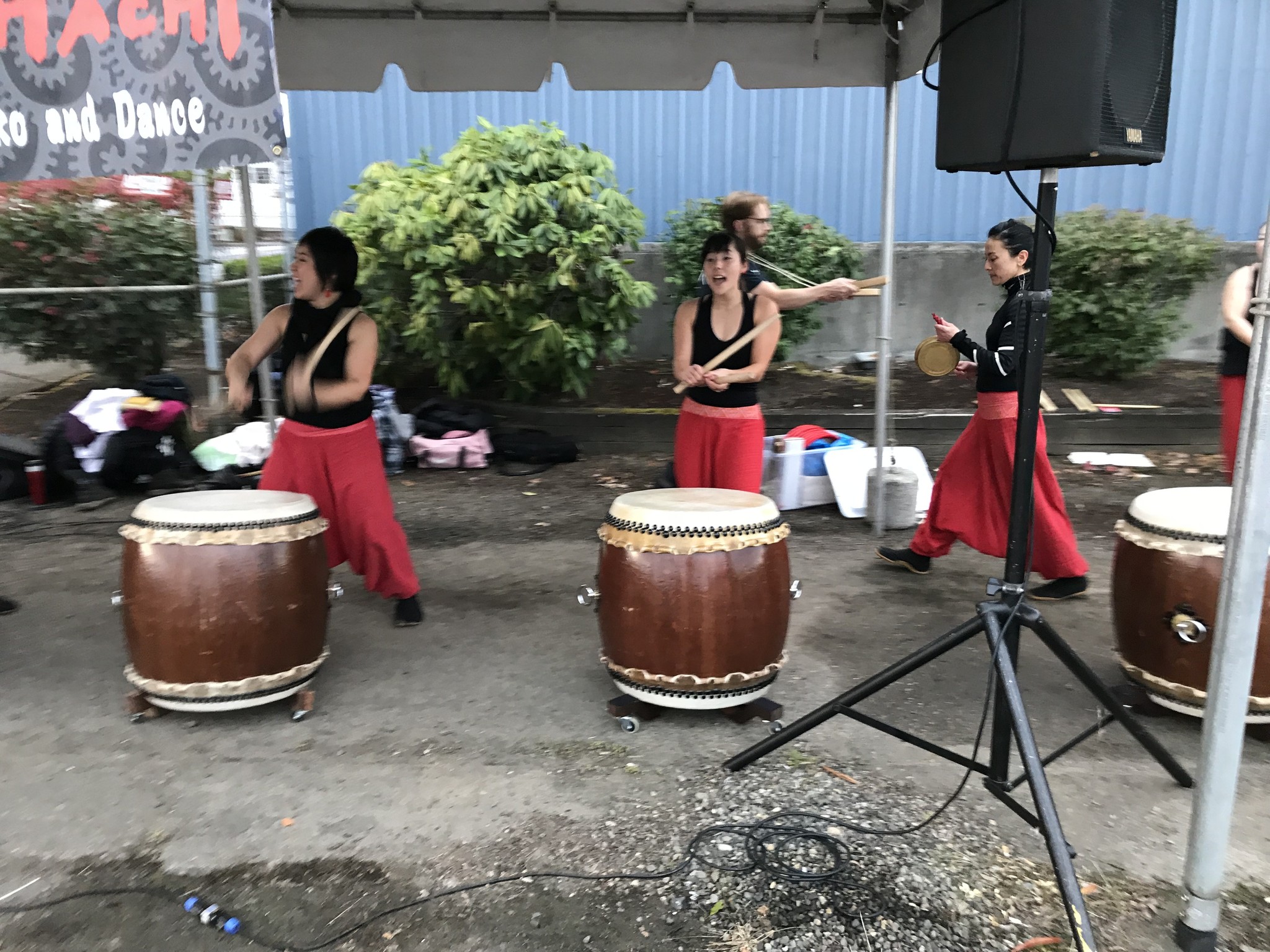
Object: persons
671 231 782 494
225 226 425 628
875 219 1090 601
652 190 860 488
1216 224 1267 486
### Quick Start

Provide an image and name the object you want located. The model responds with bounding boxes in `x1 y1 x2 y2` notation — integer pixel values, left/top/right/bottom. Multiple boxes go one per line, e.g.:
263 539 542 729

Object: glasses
741 216 769 223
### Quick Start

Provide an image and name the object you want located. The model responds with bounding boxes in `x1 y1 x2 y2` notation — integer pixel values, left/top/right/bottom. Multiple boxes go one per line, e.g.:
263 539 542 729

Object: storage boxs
761 430 867 510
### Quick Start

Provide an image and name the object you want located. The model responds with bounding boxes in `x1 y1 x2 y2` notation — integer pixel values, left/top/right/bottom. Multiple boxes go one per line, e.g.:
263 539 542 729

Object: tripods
721 162 1194 952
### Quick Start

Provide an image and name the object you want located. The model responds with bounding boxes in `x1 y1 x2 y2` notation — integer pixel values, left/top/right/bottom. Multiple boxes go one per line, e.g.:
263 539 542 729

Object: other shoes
395 597 425 630
1027 575 1087 599
875 542 930 574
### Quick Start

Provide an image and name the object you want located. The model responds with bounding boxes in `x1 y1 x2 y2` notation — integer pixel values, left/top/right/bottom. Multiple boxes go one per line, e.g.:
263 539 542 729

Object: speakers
934 0 1178 174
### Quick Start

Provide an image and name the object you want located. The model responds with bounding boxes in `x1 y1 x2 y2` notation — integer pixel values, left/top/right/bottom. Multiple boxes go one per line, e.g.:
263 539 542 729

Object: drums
592 486 792 711
119 489 329 713
914 335 960 376
1109 485 1270 727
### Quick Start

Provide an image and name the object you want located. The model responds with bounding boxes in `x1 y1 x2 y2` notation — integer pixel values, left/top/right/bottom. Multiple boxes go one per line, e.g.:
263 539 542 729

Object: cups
24 459 47 505
